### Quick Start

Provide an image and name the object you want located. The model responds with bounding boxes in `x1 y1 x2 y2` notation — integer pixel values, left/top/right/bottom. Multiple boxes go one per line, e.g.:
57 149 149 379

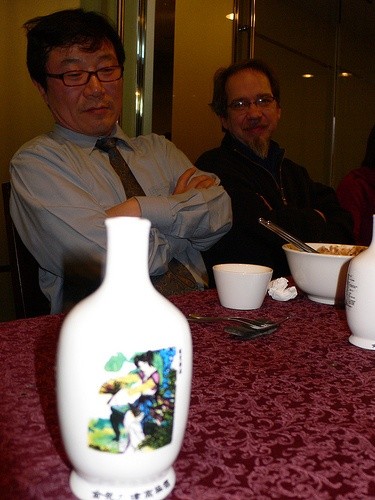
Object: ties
95 137 146 200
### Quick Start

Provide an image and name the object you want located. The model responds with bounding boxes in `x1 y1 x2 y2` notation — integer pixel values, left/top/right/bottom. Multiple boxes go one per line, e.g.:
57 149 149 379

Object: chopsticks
258 217 317 253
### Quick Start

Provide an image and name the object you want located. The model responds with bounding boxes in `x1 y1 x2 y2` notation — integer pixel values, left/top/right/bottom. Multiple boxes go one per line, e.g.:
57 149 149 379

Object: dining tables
0 272 375 500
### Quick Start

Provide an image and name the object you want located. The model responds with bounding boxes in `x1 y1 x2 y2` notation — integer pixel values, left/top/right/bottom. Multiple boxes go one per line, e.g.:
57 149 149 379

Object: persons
337 124 375 246
193 58 354 286
10 9 232 313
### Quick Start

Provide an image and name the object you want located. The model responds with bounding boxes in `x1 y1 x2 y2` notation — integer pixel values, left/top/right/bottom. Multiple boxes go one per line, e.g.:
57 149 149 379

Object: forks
185 316 290 330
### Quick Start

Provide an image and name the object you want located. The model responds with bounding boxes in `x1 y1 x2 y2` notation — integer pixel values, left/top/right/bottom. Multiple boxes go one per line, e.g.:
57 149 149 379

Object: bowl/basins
282 242 370 306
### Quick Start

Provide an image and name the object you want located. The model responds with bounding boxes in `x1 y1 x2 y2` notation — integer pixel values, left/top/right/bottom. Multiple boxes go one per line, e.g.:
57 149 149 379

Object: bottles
56 216 193 499
345 213 375 350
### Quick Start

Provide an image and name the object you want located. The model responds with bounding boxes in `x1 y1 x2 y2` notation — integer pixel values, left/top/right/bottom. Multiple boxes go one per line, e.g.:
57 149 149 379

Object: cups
214 263 274 310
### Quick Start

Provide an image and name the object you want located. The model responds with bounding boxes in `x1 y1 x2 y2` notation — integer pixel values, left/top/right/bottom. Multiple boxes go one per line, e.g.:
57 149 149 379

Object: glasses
46 65 123 87
226 96 275 110
